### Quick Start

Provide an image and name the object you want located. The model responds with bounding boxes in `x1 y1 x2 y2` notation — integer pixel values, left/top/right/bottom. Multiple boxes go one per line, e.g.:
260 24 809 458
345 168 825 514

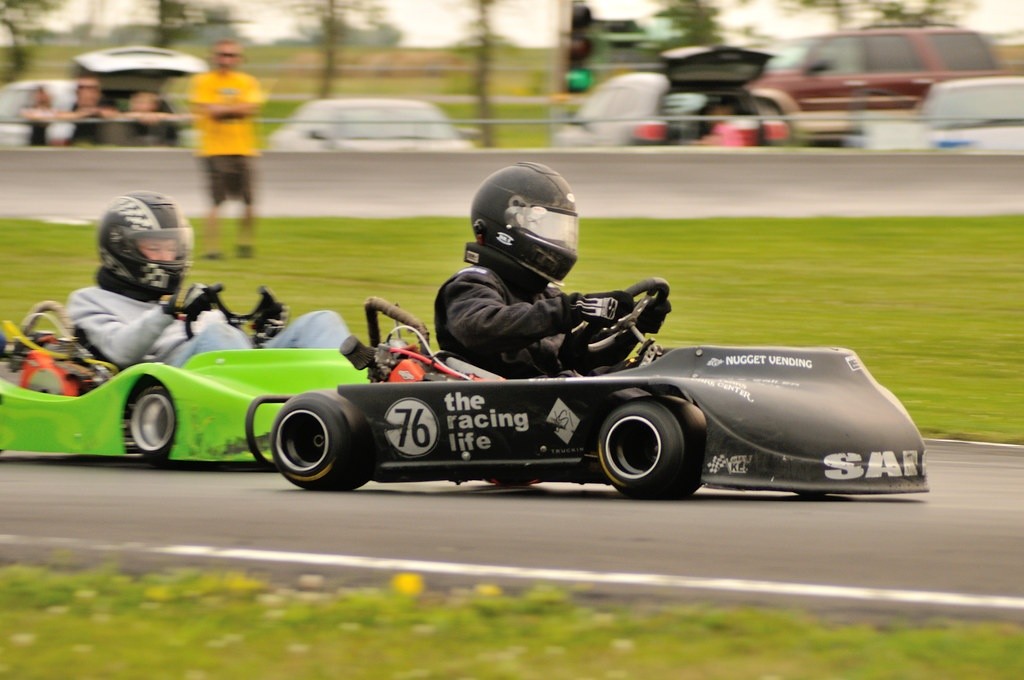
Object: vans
751 25 997 140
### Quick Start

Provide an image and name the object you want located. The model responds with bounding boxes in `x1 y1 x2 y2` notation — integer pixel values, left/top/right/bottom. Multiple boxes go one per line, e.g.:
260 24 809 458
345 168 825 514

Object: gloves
253 302 289 340
183 282 218 321
563 290 633 327
630 299 671 334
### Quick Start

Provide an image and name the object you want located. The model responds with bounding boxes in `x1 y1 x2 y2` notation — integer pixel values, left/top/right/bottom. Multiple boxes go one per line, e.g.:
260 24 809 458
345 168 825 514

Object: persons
125 89 178 146
62 191 355 377
183 39 270 262
21 83 60 146
431 159 671 384
62 79 122 146
684 101 760 148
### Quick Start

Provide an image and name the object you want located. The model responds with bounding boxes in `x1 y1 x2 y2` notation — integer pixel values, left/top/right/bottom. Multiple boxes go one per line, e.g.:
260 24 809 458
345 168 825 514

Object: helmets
94 190 194 301
472 161 581 286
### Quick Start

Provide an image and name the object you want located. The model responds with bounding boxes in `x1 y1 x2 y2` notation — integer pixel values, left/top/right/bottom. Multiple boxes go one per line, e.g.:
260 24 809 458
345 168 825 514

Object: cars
553 45 808 150
920 75 1023 153
1 46 210 147
267 96 480 152
